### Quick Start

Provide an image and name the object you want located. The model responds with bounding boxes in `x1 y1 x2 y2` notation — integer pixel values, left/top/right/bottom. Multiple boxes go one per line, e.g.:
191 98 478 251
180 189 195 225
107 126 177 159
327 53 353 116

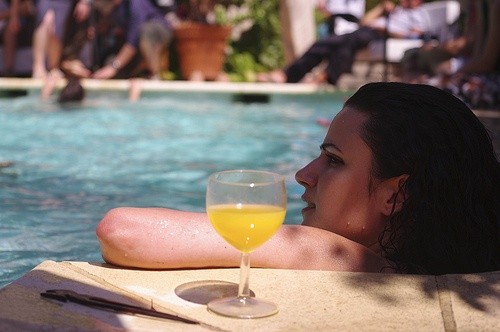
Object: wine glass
205 169 287 319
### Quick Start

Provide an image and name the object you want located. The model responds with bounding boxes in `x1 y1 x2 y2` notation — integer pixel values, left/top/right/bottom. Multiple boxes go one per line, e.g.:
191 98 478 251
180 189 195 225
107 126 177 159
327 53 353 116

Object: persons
94 82 500 275
0 0 500 114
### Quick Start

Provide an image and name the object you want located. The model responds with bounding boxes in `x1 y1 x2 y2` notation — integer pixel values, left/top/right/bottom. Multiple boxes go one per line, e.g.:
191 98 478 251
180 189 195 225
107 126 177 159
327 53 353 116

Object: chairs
362 36 426 80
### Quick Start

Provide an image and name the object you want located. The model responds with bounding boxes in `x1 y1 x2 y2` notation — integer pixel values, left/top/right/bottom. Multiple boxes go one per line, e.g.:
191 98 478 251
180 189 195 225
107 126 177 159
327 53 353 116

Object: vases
175 24 232 82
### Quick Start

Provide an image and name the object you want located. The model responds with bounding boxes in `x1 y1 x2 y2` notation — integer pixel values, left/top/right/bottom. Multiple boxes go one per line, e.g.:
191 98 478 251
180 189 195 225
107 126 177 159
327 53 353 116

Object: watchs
110 59 123 73
